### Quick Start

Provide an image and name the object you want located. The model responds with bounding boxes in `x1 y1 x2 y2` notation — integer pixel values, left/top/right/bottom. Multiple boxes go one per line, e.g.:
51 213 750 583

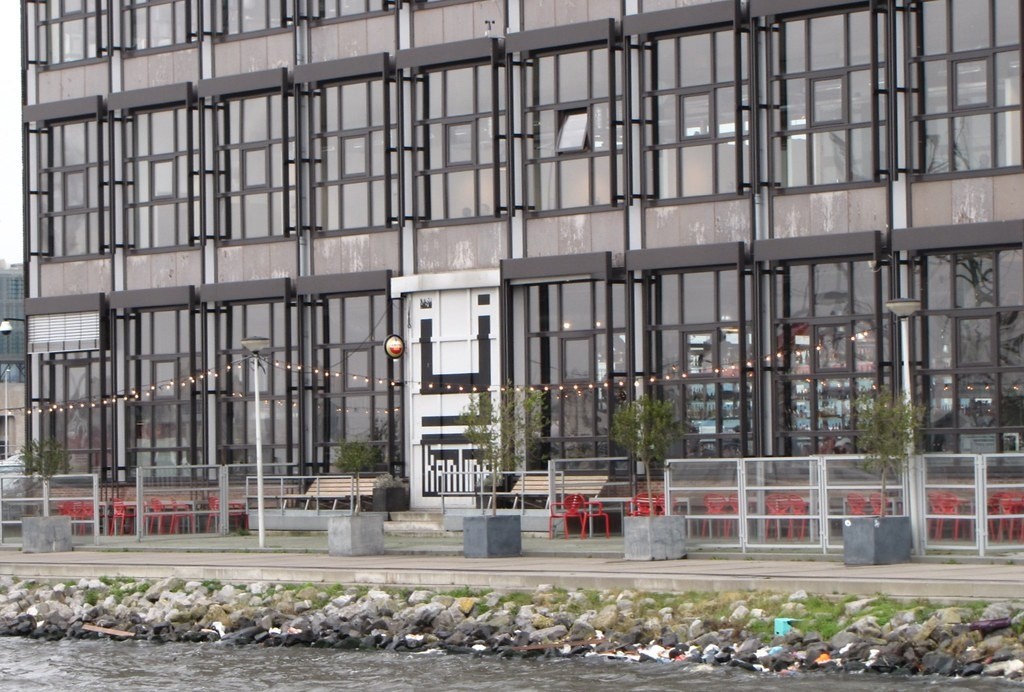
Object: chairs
548 490 1024 542
58 497 250 537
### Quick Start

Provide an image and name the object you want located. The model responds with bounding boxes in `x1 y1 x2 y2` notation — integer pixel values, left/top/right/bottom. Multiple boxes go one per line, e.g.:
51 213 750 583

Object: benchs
504 475 609 510
246 495 347 511
281 478 386 513
437 491 571 510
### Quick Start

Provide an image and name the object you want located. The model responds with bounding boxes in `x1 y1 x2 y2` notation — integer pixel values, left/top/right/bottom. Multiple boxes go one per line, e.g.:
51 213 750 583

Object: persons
820 421 852 454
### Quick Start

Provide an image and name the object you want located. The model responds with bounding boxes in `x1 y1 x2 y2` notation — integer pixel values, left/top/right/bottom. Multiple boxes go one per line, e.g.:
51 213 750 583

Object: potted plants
16 437 72 551
452 378 549 560
328 437 389 555
475 473 510 511
608 392 687 560
839 382 927 564
372 474 406 512
